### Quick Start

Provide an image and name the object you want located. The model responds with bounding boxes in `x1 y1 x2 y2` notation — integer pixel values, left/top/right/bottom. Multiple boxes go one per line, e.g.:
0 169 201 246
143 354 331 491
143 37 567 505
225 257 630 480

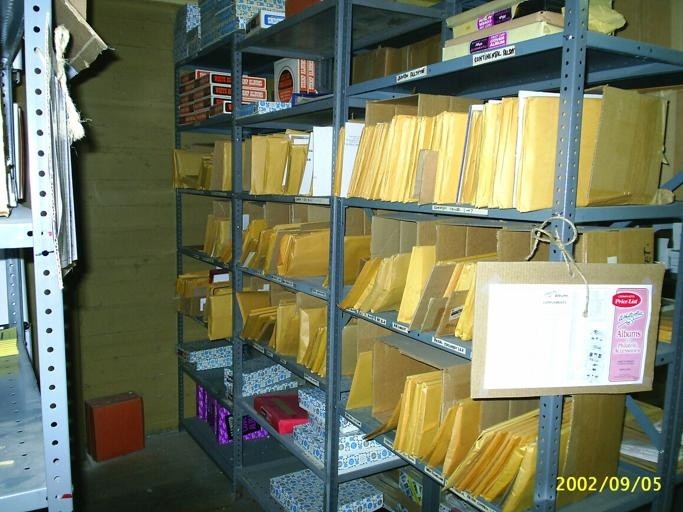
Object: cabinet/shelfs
0 0 74 512
237 1 325 511
327 1 683 509
172 31 236 510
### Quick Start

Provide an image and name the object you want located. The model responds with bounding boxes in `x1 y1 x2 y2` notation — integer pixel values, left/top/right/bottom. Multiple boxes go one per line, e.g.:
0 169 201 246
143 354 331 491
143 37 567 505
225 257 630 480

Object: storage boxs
174 1 285 62
